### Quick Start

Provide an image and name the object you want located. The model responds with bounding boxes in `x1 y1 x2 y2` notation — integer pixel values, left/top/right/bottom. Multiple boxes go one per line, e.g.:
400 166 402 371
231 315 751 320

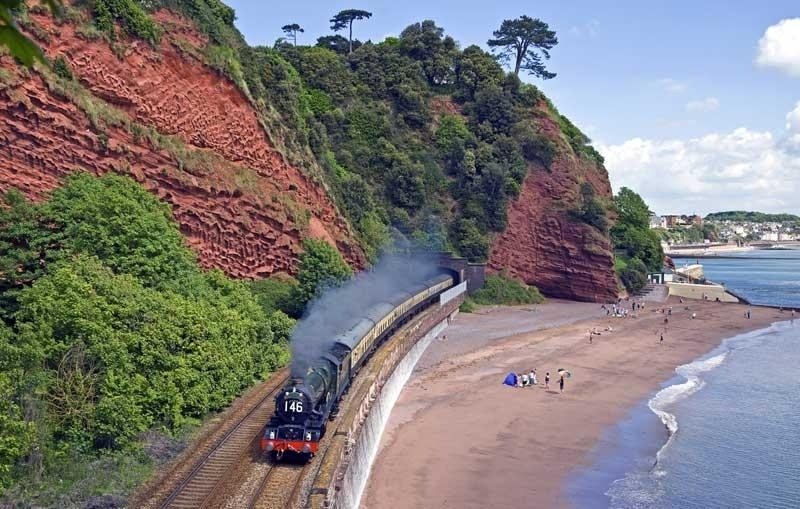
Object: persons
515 368 538 387
545 372 551 390
584 294 796 345
556 375 563 392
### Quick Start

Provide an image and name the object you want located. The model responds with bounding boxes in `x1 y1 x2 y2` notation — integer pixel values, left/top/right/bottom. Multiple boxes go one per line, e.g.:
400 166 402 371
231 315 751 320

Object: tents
503 372 517 386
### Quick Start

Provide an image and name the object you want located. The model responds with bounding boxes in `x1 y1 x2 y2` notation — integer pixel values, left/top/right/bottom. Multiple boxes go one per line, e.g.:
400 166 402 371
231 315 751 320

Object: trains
261 271 455 464
